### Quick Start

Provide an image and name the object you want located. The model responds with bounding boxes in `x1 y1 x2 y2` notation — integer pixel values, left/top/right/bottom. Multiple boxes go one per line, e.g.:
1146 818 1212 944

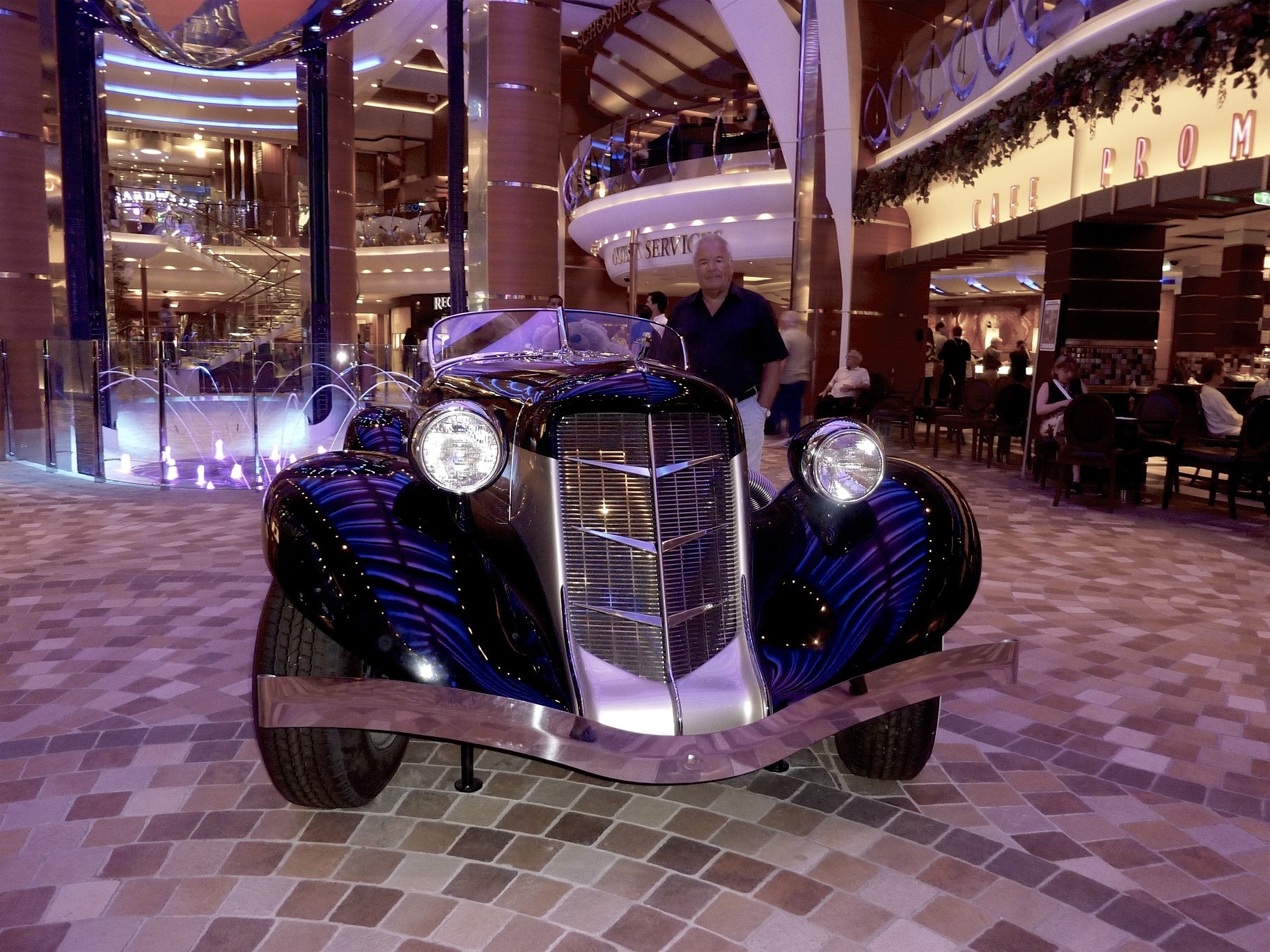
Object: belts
736 388 756 402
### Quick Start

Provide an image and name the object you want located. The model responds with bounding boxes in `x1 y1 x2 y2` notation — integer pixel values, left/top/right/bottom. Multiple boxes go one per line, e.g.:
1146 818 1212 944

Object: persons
142 208 157 223
769 311 816 448
1199 357 1244 438
660 234 788 474
109 172 117 220
543 291 686 370
815 350 871 419
1035 353 1089 493
924 320 1032 408
158 297 178 367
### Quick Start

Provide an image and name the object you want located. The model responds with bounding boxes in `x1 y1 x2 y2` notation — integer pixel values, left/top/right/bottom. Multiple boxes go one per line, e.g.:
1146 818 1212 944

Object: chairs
858 376 1270 519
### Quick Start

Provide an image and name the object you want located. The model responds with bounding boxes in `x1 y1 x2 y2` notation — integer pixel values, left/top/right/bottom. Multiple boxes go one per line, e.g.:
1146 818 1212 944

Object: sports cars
251 306 1021 811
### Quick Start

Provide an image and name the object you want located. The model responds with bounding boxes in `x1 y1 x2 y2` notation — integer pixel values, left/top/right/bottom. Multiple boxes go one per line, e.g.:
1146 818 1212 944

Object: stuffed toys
451 311 633 357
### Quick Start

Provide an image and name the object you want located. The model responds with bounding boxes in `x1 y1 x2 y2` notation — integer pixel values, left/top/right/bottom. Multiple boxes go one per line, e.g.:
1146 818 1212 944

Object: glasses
845 355 859 359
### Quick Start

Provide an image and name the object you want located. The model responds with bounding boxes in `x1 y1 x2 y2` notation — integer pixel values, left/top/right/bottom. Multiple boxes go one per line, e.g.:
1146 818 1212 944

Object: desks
1111 414 1139 489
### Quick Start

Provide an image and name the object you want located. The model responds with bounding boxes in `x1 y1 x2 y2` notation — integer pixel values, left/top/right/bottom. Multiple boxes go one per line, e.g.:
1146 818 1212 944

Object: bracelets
853 385 856 390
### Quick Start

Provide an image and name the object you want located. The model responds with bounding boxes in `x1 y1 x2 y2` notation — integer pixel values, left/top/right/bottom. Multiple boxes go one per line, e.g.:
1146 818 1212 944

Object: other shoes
781 437 793 448
1070 482 1079 493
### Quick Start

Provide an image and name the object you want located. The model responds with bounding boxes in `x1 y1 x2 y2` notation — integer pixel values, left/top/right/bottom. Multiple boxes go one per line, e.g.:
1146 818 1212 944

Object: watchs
761 407 771 418
